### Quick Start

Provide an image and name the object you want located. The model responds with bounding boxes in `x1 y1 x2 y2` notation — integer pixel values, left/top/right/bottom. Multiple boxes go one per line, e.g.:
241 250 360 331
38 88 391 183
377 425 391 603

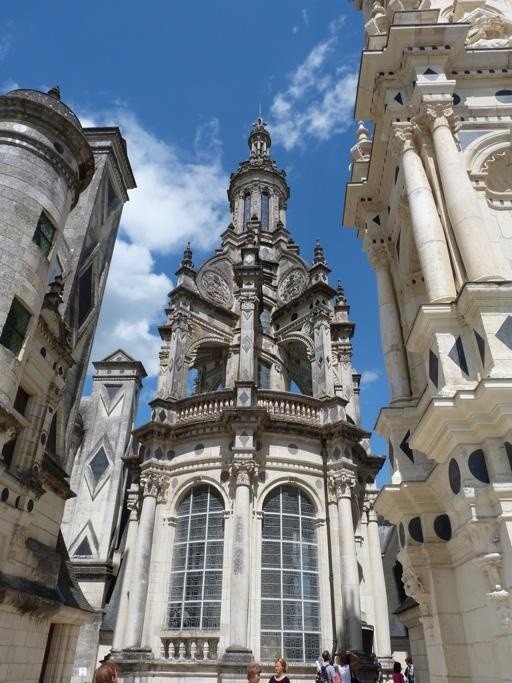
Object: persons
337 649 361 682
318 650 336 682
403 656 415 683
369 653 383 683
391 661 405 683
268 656 290 683
246 663 262 683
94 661 118 683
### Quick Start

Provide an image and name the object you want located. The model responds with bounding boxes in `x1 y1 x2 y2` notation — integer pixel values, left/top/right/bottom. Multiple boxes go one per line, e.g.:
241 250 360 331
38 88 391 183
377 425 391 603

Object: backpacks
315 663 331 682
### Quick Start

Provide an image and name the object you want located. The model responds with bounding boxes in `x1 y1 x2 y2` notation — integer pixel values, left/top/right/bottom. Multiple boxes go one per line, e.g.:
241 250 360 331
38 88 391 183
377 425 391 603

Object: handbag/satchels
350 663 361 682
